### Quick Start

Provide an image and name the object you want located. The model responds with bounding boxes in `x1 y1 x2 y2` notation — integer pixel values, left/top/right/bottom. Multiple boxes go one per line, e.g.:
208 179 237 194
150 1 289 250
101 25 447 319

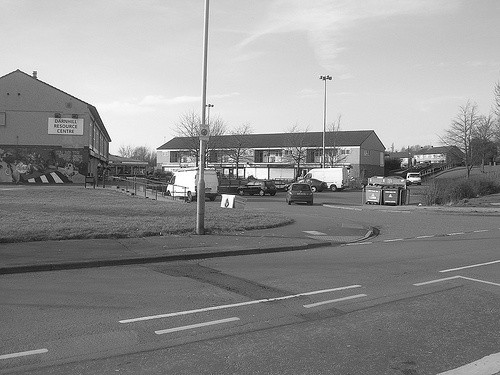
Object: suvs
235 179 277 196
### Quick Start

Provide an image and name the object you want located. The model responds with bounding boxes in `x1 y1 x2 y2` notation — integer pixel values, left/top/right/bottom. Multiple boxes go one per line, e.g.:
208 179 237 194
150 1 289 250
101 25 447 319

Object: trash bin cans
383 185 399 204
365 184 383 204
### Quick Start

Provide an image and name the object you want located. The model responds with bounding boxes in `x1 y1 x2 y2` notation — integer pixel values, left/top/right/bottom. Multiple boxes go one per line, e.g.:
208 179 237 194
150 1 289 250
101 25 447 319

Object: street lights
318 75 332 168
206 103 214 167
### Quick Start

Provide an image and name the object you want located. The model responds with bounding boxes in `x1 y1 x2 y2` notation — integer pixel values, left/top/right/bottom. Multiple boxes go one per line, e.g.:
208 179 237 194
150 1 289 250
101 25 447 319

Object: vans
406 173 422 185
166 167 219 201
297 167 349 192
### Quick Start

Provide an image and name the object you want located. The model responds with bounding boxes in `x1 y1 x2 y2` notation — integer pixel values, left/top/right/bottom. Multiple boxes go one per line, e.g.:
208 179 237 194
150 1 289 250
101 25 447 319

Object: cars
271 180 287 191
384 176 412 186
286 183 314 205
285 178 327 193
